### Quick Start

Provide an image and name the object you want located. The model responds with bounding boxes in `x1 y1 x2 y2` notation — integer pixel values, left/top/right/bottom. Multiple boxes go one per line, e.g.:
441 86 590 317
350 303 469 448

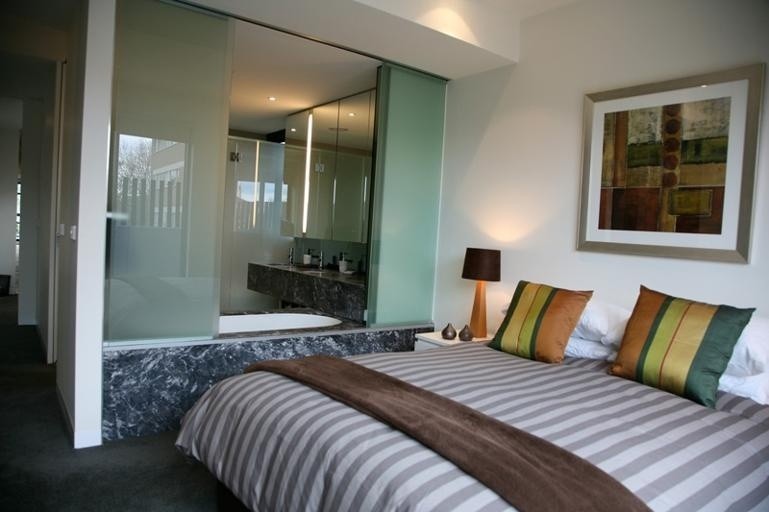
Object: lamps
461 248 501 338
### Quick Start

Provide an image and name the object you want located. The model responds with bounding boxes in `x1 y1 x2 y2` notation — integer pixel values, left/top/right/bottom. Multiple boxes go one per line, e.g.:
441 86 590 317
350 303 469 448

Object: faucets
288 247 294 264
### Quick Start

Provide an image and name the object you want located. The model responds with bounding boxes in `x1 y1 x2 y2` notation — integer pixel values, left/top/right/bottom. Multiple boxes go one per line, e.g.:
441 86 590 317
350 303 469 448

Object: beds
174 340 768 512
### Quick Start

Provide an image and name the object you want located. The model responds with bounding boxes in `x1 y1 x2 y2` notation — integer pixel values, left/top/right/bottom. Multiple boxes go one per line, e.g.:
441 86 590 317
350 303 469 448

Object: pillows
501 297 632 342
600 317 764 378
487 280 594 362
607 285 756 408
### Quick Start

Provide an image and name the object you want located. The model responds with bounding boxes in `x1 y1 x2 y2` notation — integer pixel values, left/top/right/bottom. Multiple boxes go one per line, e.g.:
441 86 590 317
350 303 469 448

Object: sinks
268 264 320 269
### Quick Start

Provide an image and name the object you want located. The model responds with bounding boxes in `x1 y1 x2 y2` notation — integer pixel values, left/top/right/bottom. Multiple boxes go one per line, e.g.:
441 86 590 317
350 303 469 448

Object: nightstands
413 332 492 351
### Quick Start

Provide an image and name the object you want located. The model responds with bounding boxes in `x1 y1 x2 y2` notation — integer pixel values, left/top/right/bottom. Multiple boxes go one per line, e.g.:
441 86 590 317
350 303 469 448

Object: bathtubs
219 313 342 334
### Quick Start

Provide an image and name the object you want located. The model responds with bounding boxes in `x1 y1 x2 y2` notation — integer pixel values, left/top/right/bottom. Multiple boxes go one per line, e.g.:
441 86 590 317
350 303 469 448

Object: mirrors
280 88 376 243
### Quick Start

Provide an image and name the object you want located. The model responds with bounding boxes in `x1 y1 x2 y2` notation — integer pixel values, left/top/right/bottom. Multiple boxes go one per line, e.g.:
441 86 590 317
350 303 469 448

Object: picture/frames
575 62 765 264
